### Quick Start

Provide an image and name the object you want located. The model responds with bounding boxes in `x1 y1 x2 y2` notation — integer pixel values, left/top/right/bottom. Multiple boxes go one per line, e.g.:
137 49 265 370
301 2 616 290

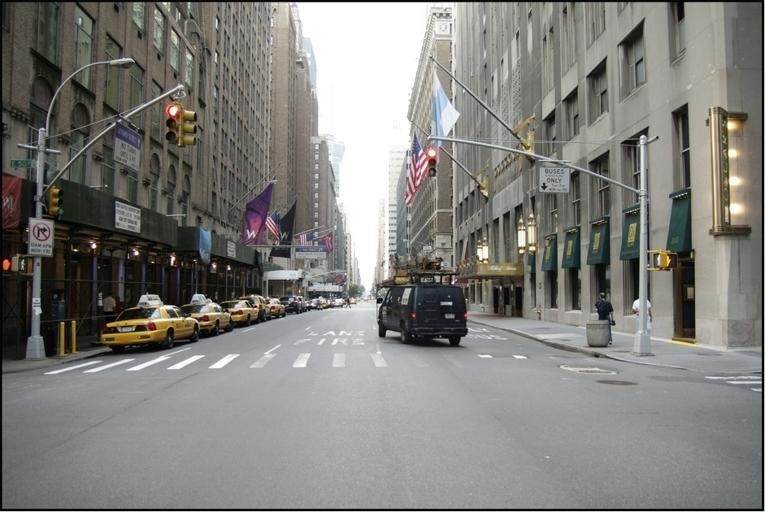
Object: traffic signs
10 158 35 169
539 167 569 193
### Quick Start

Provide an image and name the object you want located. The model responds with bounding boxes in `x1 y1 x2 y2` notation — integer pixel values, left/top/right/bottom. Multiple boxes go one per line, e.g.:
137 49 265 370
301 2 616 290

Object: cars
98 292 200 350
176 293 233 337
243 293 362 319
217 297 264 327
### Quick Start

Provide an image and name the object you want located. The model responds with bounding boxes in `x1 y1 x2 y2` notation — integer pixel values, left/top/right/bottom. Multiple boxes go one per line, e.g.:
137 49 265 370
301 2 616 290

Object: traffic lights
12 254 28 274
654 254 677 267
180 107 199 148
1 253 11 274
164 102 183 146
344 273 347 279
426 147 437 179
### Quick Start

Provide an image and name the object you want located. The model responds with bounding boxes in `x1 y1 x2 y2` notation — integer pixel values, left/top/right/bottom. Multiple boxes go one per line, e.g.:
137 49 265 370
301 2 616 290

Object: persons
100 288 354 325
595 292 616 346
632 290 654 336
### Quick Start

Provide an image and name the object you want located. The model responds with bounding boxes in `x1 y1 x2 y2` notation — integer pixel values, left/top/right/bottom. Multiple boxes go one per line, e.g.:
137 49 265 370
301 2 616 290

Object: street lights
401 238 432 247
24 56 136 362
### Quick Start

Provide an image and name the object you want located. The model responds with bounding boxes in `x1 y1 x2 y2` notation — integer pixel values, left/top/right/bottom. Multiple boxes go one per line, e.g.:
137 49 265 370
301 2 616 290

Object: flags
239 175 334 257
405 132 431 207
430 68 461 148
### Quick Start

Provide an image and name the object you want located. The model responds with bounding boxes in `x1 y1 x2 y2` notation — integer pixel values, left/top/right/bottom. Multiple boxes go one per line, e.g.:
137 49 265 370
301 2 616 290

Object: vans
375 283 469 346
